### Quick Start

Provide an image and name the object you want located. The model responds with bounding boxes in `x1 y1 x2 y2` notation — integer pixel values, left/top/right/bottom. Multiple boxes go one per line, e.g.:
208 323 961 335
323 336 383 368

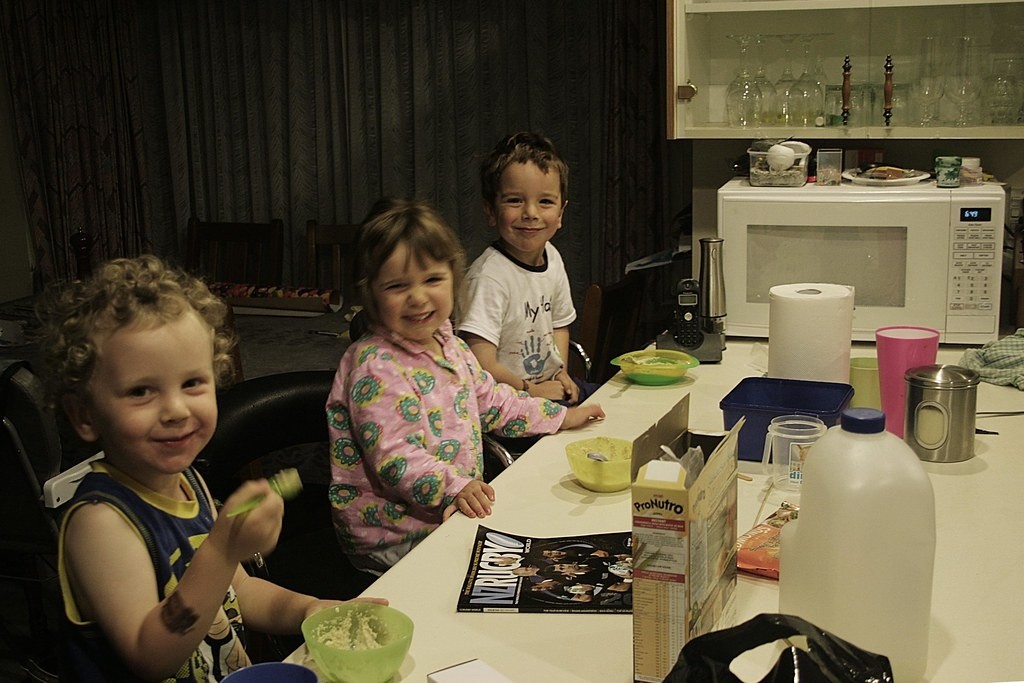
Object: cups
934 156 980 188
903 364 981 462
823 83 910 127
982 59 1024 125
763 416 827 492
875 326 941 440
850 357 882 414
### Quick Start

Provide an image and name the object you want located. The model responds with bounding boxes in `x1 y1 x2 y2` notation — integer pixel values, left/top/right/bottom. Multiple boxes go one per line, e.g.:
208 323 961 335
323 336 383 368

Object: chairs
181 215 668 602
0 360 259 683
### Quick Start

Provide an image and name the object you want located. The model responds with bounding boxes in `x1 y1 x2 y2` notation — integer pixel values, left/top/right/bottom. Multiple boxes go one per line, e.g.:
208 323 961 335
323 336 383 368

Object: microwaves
716 176 1006 344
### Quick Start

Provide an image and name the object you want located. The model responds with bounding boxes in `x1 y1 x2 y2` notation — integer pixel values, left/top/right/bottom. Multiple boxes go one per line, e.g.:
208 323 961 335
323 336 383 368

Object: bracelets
522 379 529 392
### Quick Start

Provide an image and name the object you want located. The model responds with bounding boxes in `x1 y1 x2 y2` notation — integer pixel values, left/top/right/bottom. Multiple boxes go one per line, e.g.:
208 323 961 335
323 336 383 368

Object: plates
842 169 931 185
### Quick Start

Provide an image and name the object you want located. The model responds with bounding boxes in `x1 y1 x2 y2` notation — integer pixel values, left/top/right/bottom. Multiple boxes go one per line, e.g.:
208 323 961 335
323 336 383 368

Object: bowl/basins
219 663 318 683
747 147 813 187
565 438 635 492
611 349 699 386
300 603 414 683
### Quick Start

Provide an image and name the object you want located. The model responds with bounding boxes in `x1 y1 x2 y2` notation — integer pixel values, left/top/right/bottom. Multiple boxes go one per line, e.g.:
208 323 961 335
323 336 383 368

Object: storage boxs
630 390 746 682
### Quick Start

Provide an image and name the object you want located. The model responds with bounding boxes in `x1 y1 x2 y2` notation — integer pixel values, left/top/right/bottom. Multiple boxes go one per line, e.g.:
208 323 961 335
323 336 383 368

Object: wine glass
724 32 831 128
914 35 983 127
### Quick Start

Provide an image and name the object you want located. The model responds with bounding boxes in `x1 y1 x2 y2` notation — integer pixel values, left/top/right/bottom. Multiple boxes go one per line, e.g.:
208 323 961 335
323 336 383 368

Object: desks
0 285 359 405
285 326 1022 682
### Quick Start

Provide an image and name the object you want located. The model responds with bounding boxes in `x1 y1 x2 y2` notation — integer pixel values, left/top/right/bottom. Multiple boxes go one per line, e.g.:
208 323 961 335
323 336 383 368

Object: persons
452 127 604 456
327 190 605 583
21 255 388 683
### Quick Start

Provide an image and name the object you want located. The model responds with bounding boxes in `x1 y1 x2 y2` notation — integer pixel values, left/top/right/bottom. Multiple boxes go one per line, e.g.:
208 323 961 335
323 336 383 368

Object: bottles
778 408 935 683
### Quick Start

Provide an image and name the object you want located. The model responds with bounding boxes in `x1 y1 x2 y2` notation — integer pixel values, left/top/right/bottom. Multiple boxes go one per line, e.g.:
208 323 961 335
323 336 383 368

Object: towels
958 327 1024 390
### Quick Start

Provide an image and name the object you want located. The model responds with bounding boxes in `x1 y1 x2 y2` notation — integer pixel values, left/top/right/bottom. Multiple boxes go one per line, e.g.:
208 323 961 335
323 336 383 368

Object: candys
207 281 334 305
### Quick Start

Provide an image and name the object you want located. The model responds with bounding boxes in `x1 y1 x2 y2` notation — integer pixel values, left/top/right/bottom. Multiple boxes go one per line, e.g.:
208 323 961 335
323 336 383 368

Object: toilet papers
768 282 855 384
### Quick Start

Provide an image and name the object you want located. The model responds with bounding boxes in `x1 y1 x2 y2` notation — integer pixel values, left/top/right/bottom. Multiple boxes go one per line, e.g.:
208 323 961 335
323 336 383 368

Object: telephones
656 278 722 364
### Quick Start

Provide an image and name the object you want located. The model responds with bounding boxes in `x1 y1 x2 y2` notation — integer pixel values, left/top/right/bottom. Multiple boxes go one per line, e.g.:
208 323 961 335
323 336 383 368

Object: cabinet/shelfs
666 1 1023 142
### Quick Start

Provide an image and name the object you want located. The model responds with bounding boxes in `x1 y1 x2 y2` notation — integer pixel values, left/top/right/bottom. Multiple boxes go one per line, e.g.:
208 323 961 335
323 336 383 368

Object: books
457 523 633 615
